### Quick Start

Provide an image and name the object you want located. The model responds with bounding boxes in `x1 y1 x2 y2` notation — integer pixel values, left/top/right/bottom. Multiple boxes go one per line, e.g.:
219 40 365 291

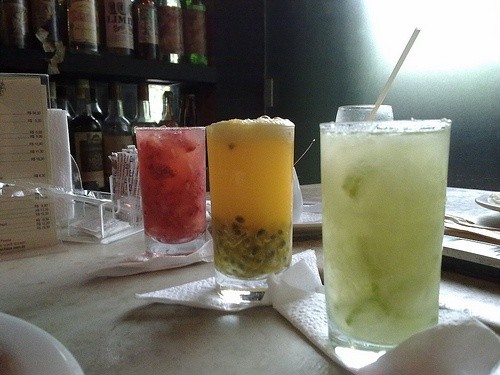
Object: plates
292 212 321 236
475 192 500 210
0 312 84 375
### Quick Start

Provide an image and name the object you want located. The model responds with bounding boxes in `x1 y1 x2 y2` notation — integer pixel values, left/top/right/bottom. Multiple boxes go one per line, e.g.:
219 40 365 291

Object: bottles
0 0 209 64
47 72 201 193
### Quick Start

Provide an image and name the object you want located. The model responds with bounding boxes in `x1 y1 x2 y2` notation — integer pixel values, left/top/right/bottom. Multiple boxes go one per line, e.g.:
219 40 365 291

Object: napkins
135 247 320 316
257 277 500 375
81 239 214 279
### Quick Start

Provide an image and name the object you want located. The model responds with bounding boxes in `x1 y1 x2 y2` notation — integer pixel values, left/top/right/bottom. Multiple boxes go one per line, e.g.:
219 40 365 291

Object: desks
0 183 500 375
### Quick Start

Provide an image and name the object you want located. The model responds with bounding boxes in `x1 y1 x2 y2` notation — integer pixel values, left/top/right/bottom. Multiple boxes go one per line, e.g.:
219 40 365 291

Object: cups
319 119 451 349
337 105 393 124
137 126 207 260
206 124 297 304
